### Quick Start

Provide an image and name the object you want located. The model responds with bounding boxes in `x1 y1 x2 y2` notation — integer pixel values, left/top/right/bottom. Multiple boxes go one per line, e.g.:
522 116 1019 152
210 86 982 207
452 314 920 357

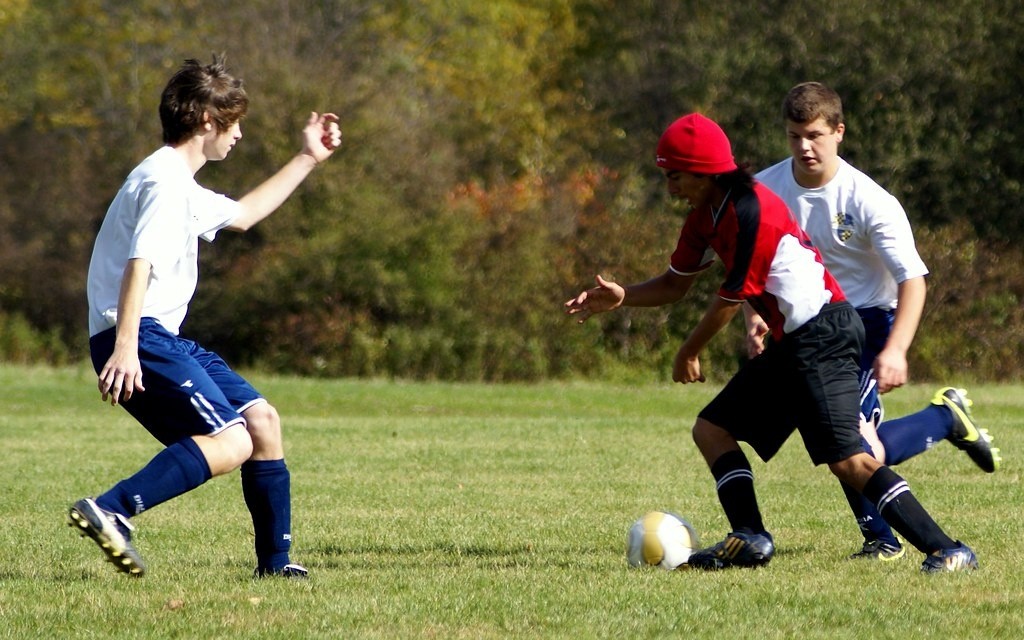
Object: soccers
625 510 700 570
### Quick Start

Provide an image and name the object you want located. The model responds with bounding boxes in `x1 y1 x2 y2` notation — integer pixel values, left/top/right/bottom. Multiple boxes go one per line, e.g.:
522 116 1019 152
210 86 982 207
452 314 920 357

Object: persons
69 48 342 579
564 112 977 574
739 82 1001 561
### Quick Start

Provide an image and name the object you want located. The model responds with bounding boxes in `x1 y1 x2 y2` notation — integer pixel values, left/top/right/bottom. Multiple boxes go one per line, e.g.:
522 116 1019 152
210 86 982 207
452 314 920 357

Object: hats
656 113 738 174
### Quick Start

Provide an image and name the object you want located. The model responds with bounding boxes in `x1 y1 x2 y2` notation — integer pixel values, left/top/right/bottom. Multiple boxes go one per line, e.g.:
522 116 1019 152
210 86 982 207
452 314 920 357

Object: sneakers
849 537 906 562
251 563 308 580
688 530 773 571
931 386 1002 473
69 498 145 578
919 539 978 575
